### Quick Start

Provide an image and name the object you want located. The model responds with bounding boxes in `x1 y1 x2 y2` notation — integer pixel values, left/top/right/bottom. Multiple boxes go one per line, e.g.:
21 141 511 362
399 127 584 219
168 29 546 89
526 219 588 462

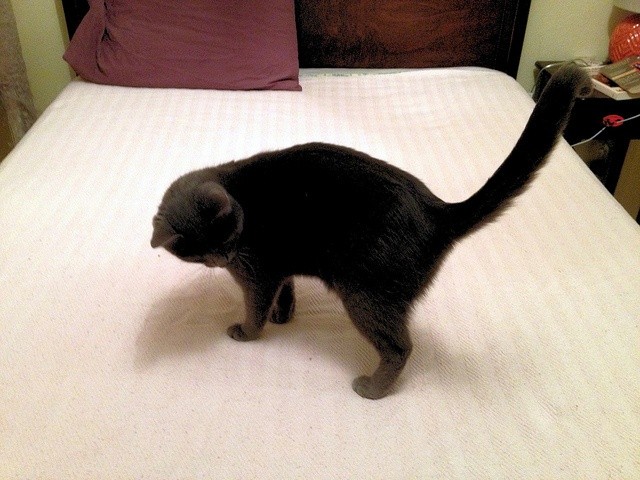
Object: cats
151 59 594 401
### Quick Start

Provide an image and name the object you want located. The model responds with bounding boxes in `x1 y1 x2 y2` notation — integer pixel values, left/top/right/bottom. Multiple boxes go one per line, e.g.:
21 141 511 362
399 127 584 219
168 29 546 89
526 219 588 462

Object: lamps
608 0 640 63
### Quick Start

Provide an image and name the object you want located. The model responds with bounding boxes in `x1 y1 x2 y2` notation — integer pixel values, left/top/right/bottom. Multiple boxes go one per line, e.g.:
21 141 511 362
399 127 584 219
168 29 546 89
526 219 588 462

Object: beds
0 0 640 477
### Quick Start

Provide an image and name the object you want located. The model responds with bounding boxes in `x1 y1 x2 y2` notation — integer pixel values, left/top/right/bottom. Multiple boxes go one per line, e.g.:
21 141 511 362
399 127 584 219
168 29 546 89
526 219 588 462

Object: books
584 68 640 101
600 64 640 96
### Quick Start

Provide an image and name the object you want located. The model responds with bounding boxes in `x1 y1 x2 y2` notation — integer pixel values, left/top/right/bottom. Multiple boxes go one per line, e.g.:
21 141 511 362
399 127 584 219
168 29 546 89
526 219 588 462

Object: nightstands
535 59 640 196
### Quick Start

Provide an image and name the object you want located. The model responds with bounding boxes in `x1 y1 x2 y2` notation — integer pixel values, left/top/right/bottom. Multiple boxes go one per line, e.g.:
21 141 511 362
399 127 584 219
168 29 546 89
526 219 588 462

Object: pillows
61 0 305 93
